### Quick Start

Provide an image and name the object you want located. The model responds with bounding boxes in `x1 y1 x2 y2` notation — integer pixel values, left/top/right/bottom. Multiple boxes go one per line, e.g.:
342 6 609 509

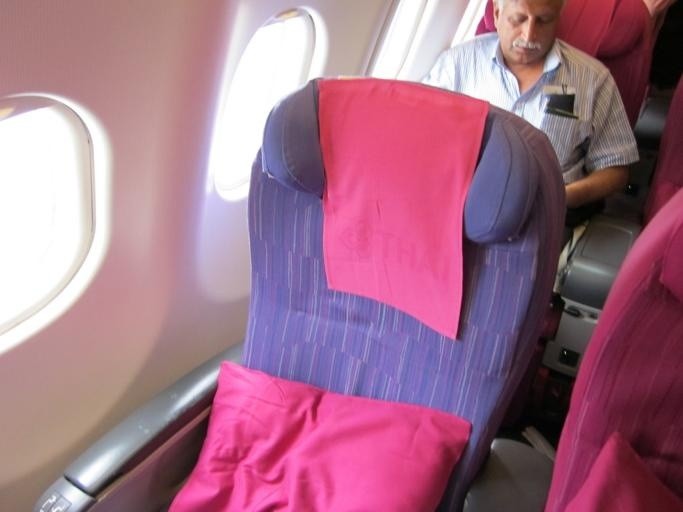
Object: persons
419 1 643 257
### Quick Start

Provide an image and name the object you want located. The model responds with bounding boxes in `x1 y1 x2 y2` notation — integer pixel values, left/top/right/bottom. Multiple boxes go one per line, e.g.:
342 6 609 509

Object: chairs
30 0 683 512
